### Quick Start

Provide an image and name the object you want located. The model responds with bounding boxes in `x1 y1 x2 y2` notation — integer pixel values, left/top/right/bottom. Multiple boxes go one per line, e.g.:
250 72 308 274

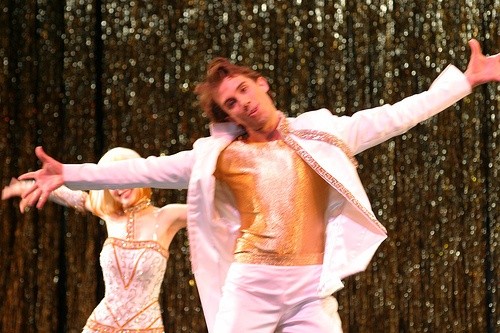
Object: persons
16 37 500 333
2 145 189 332
